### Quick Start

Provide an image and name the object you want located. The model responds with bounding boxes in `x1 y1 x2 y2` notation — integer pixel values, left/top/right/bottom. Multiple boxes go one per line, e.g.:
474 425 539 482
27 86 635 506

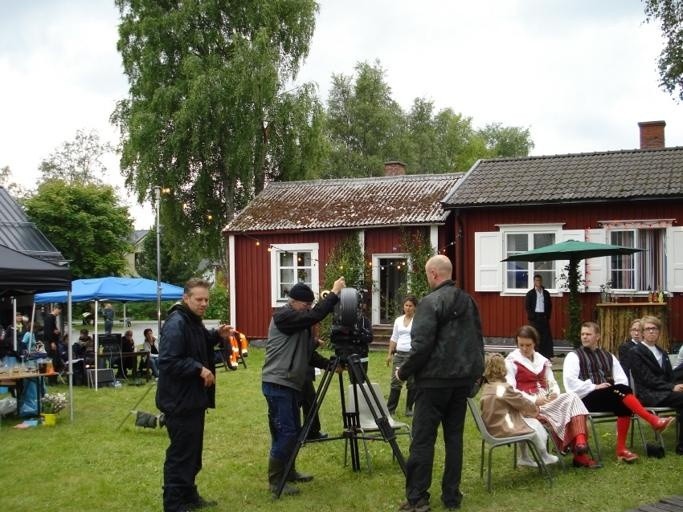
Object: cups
36 359 52 374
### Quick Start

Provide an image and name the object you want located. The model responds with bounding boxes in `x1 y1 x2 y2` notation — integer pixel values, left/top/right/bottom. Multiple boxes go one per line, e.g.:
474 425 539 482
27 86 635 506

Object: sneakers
442 495 462 511
538 454 560 466
396 496 433 512
269 470 315 498
517 456 539 468
162 494 220 512
306 431 329 440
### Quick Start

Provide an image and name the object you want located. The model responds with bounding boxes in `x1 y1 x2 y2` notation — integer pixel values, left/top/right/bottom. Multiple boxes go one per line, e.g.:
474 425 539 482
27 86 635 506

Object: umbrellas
501 240 644 347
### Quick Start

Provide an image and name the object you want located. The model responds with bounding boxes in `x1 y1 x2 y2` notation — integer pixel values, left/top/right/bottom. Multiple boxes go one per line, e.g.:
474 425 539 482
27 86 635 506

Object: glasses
644 326 660 332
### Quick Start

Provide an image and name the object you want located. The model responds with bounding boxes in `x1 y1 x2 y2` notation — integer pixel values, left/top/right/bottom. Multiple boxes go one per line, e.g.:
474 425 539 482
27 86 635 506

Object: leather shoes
653 414 676 438
617 450 640 464
571 443 605 469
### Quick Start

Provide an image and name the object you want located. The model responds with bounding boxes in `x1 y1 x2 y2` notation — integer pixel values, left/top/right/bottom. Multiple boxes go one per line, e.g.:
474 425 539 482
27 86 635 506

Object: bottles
647 289 664 302
600 287 615 303
5 354 34 376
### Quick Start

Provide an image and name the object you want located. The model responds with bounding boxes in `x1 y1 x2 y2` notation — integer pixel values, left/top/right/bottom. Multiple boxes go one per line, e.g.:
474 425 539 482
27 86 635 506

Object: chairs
342 381 413 470
0 316 249 431
462 353 682 495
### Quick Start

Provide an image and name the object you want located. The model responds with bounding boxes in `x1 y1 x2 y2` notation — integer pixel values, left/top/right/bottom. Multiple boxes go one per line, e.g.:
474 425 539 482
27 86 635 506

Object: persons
301 308 343 439
398 253 485 510
617 317 645 378
672 342 682 370
154 277 235 512
478 351 559 468
385 295 418 418
0 300 159 418
627 315 682 458
260 275 347 504
504 324 606 469
563 319 674 463
524 275 554 362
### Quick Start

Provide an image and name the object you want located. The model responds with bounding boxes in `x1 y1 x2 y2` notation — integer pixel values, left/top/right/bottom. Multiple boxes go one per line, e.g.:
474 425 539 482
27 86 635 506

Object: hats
286 281 317 303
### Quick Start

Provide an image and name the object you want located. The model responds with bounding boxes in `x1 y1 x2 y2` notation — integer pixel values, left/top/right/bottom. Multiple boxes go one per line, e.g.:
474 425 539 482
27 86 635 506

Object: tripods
270 324 408 498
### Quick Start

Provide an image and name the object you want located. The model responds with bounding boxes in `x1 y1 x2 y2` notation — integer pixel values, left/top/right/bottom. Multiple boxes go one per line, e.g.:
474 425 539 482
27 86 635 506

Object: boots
386 388 401 417
405 388 417 417
